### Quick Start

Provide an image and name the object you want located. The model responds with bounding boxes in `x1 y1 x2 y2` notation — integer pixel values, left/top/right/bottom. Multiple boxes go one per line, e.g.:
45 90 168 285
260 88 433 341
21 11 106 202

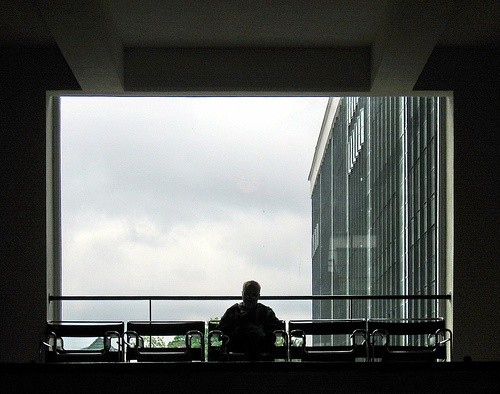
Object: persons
214 281 287 360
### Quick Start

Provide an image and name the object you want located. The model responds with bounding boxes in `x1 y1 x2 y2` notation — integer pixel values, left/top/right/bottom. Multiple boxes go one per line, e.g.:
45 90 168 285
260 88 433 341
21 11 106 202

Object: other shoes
261 333 276 351
257 352 277 361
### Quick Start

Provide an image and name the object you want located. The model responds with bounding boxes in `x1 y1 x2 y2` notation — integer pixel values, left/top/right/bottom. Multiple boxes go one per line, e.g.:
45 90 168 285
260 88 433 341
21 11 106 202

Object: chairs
367 318 452 361
123 322 205 362
208 321 288 361
38 323 124 361
289 318 366 361
